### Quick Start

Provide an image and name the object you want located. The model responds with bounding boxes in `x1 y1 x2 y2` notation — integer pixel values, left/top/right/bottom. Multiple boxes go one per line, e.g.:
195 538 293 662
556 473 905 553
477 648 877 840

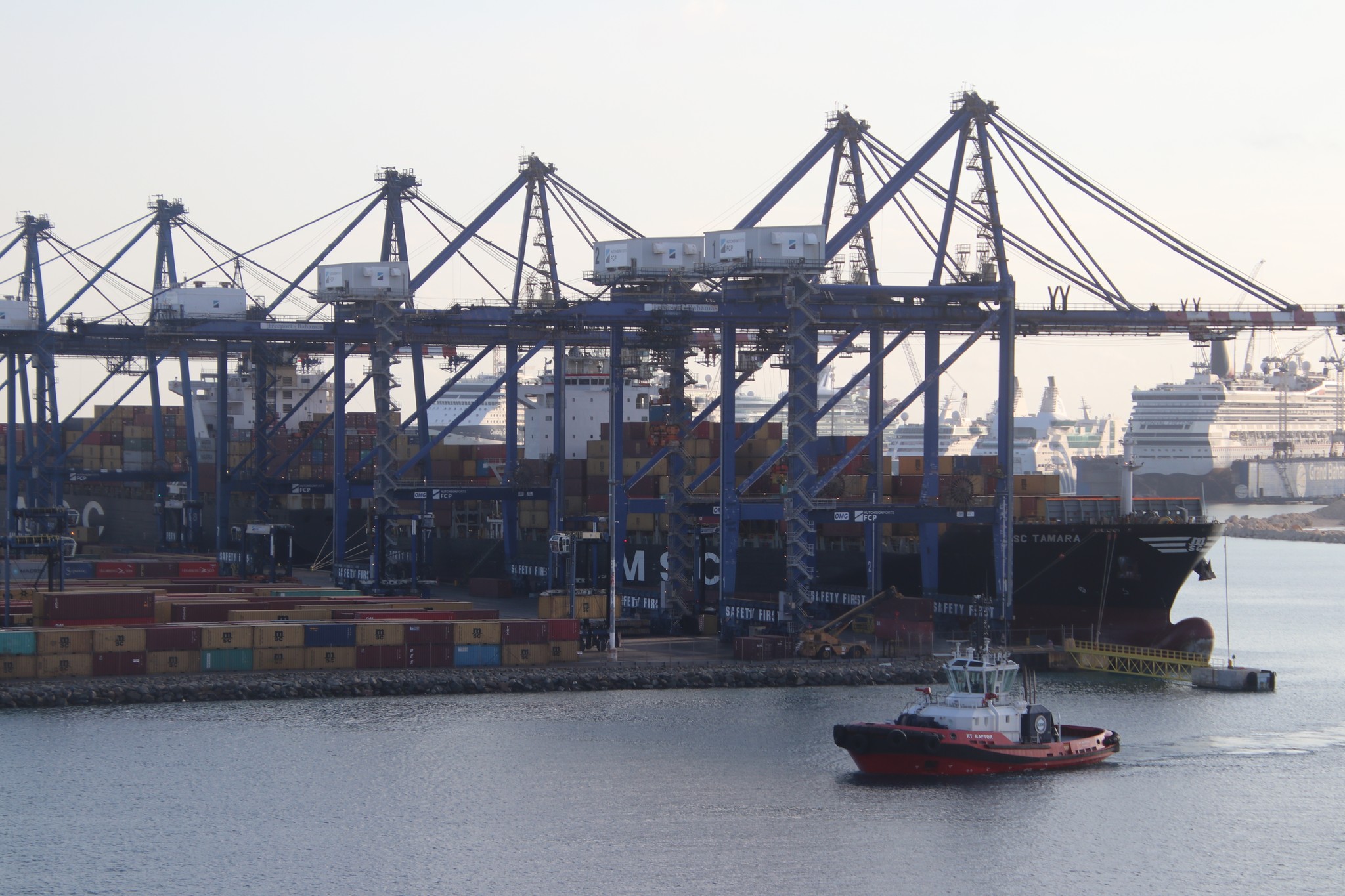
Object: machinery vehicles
797 585 901 660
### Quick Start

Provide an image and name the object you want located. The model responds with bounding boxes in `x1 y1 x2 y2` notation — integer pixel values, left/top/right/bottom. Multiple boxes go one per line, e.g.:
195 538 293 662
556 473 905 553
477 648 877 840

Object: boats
657 363 900 451
974 377 1122 499
885 370 986 495
425 375 536 445
833 638 1121 778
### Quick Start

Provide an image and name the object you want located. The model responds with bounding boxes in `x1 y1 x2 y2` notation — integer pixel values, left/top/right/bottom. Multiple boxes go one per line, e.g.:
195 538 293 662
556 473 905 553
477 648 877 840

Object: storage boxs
0 392 1061 683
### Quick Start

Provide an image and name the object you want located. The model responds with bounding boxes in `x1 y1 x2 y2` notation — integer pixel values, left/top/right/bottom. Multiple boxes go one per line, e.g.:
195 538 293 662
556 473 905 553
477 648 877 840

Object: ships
1072 326 1345 501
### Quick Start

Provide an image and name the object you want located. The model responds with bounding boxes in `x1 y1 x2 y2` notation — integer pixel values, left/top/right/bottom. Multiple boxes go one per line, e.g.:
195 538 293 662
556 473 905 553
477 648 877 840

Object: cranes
0 91 1345 645
